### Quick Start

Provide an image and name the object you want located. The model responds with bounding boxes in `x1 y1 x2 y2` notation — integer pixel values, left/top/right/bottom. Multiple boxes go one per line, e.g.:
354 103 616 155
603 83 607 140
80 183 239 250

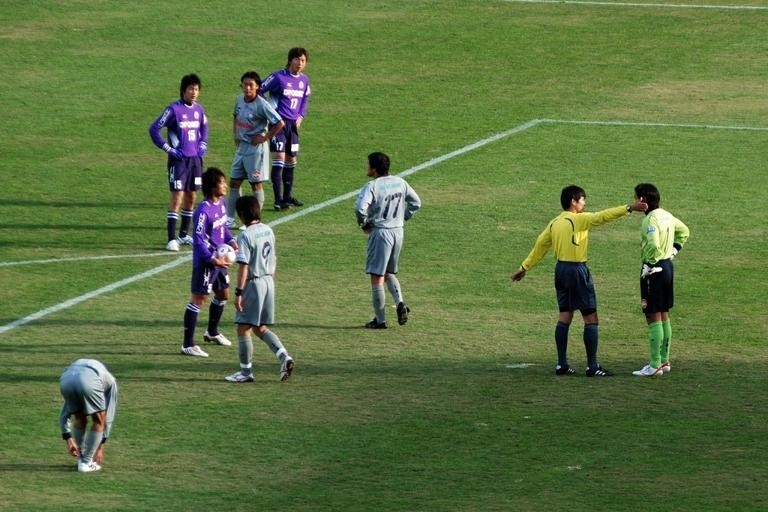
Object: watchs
627 205 632 213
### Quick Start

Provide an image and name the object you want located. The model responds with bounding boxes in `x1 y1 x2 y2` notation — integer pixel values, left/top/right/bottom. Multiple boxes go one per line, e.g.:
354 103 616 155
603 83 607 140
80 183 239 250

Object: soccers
215 243 236 266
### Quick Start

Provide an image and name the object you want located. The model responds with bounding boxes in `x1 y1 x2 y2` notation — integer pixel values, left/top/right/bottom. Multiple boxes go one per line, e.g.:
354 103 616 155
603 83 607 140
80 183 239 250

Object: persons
60 359 117 471
181 167 238 357
226 71 285 231
355 152 422 329
632 183 690 379
149 74 209 252
224 195 294 383
511 184 648 377
258 46 310 210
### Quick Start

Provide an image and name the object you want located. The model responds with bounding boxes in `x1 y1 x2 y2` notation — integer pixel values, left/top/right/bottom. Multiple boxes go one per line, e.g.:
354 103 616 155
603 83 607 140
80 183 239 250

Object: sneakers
366 317 387 330
396 302 409 325
224 371 254 382
585 366 614 376
76 460 102 471
202 330 233 346
273 203 294 211
181 345 209 357
632 365 663 376
555 366 579 376
239 225 247 230
280 356 295 381
225 220 238 229
660 361 671 372
178 236 195 245
166 240 179 251
288 198 304 206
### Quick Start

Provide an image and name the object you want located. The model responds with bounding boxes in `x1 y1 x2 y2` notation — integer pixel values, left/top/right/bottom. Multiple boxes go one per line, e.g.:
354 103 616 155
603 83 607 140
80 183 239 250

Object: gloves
199 141 208 156
163 143 182 159
